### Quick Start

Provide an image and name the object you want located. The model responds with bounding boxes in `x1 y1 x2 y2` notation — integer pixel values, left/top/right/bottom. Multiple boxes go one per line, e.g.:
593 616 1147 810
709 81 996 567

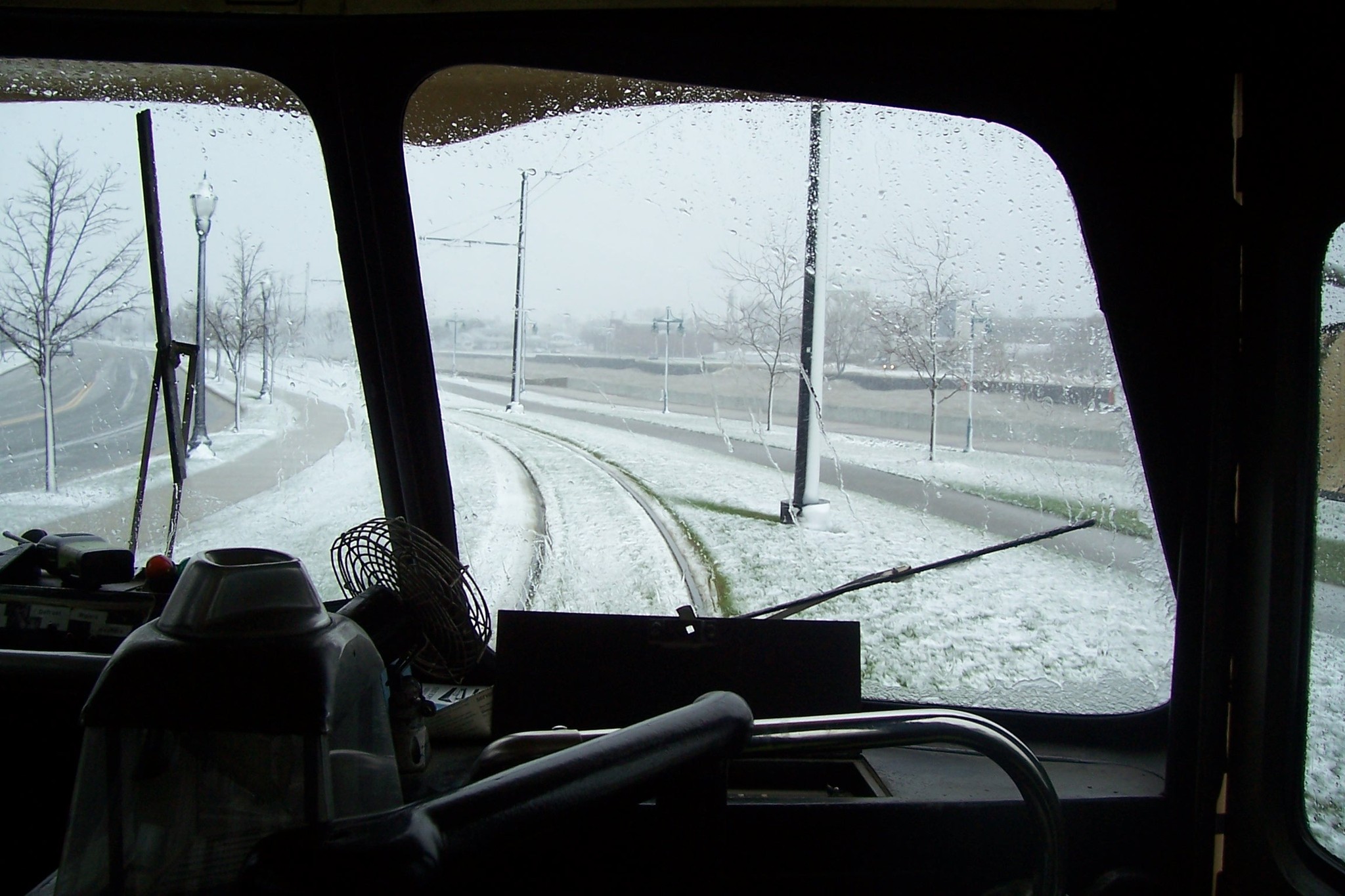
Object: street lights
190 168 222 460
445 319 468 377
946 302 994 452
260 271 275 398
651 309 688 415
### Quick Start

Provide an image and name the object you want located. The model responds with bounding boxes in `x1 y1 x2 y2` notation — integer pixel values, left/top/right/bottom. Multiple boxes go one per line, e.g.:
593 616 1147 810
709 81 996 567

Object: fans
330 516 492 772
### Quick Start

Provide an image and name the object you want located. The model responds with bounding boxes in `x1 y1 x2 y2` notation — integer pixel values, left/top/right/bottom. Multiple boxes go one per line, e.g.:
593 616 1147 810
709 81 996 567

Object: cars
53 334 72 357
